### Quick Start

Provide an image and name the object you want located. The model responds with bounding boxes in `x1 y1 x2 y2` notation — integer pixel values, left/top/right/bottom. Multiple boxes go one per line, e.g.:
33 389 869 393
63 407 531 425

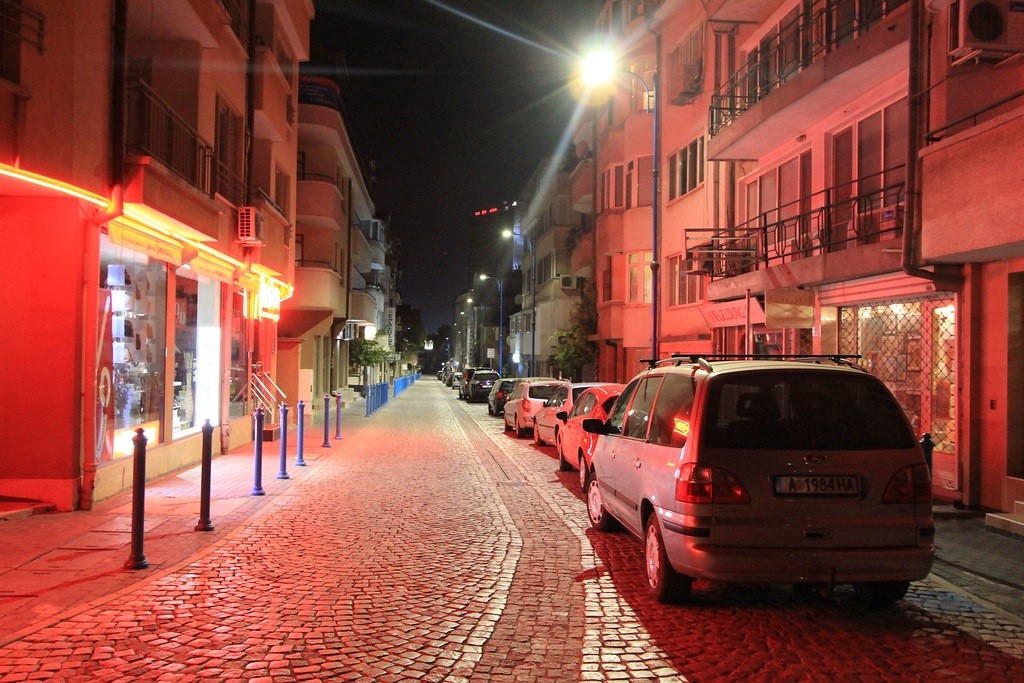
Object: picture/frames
882 314 921 373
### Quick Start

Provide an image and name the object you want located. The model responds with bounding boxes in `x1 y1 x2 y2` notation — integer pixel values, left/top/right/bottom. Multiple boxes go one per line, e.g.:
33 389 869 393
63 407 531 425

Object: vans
581 351 937 604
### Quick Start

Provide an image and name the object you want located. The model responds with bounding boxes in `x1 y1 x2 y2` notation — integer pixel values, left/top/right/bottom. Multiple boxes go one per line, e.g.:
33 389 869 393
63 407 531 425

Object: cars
436 365 557 417
532 381 621 454
555 384 629 493
503 377 572 438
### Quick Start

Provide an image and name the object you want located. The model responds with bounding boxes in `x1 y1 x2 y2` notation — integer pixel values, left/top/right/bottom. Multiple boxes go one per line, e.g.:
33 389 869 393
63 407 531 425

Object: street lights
467 297 484 367
502 228 537 378
582 45 661 362
479 274 504 378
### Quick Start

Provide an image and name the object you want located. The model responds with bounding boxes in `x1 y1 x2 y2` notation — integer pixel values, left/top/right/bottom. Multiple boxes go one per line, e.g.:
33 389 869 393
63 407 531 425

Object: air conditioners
367 219 382 242
791 229 826 260
686 246 713 275
856 205 903 246
558 335 572 346
947 0 1024 59
238 206 264 245
559 274 576 289
